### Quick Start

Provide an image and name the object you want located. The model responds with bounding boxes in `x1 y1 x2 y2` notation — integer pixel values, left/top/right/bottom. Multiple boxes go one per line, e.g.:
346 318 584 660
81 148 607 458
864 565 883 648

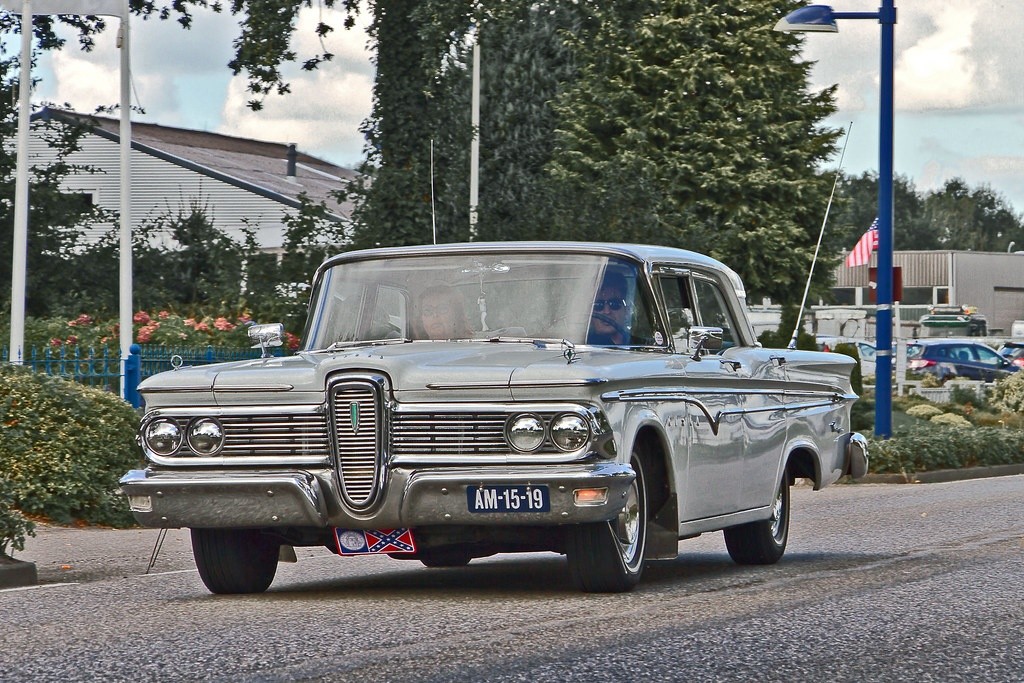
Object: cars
116 240 870 594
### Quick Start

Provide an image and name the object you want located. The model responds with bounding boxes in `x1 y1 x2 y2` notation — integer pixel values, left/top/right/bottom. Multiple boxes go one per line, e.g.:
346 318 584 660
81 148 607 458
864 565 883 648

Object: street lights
773 3 895 437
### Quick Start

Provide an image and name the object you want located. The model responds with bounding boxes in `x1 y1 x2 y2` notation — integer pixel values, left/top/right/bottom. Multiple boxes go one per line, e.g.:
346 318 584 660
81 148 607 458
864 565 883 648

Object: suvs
991 341 1024 370
907 338 1021 384
789 333 876 377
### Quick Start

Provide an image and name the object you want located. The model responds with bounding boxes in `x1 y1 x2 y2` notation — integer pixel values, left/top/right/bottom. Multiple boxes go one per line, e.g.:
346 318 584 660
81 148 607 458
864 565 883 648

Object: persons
416 285 472 340
582 270 648 346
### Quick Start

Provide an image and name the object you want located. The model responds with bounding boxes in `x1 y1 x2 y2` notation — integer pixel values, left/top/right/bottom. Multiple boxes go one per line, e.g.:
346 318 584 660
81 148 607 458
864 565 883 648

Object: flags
844 217 879 268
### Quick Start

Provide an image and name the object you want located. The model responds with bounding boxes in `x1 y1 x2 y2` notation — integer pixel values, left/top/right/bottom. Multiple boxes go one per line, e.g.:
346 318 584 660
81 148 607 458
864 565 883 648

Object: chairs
959 351 969 360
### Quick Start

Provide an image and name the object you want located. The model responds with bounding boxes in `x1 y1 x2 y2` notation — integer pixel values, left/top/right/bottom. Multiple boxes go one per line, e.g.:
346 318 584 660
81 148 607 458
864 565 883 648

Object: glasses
593 298 627 312
421 304 450 314
